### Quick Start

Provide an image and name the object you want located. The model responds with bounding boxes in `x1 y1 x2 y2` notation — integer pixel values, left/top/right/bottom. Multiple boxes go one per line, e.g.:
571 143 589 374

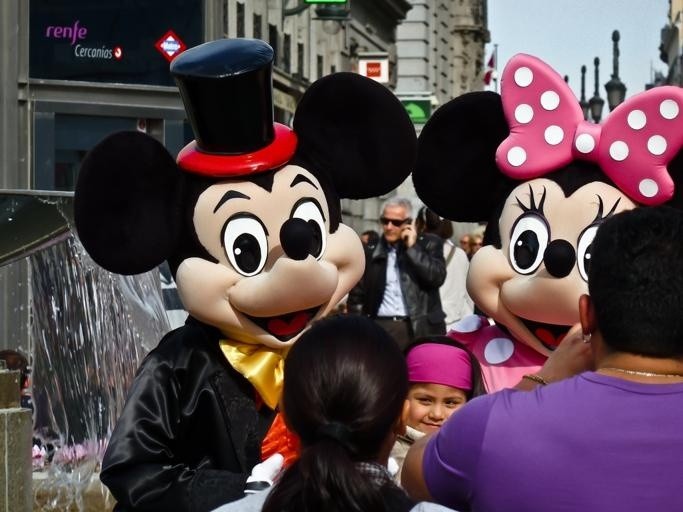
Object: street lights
577 65 589 122
607 29 626 111
589 55 604 123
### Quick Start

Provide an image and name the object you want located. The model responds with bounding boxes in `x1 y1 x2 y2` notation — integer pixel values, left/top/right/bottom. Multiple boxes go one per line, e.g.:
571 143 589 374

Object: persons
211 314 460 512
400 203 683 512
335 197 487 493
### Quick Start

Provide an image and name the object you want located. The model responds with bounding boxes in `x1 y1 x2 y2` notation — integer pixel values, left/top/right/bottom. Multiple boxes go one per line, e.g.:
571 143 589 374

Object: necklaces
593 366 681 378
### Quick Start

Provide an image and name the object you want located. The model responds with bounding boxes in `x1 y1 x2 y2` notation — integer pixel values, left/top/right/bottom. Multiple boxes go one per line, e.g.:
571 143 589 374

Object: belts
377 316 405 321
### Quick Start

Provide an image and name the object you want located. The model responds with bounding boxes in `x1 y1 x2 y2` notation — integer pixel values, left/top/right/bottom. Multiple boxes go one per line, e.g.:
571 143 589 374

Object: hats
404 343 471 391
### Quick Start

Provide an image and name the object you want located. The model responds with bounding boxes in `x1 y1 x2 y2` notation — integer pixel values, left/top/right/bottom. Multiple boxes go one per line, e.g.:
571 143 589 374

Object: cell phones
404 220 411 241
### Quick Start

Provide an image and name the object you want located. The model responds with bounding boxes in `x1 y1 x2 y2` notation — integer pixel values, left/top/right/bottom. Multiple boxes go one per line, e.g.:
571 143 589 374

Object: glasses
379 217 407 226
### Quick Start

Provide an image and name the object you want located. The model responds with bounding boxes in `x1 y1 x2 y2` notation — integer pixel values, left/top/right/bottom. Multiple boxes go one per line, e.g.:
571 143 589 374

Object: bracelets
521 374 547 385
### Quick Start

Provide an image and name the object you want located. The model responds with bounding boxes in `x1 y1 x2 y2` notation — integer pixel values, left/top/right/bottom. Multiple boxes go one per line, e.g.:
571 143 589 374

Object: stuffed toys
70 36 420 512
411 51 683 396
